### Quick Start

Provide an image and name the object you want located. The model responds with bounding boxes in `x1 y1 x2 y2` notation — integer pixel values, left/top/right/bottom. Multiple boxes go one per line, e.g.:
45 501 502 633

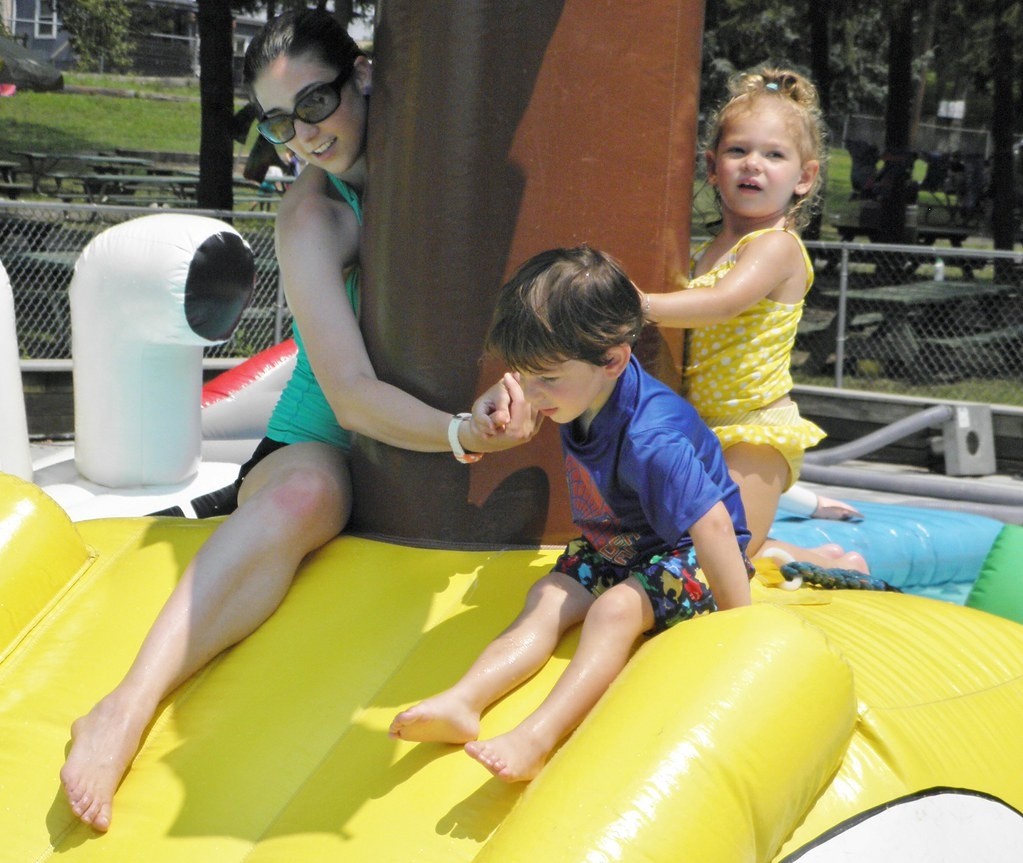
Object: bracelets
646 294 650 316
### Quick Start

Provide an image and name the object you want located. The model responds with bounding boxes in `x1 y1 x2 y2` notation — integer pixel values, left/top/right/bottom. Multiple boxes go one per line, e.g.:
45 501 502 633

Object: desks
801 279 1013 383
10 150 154 194
45 172 200 221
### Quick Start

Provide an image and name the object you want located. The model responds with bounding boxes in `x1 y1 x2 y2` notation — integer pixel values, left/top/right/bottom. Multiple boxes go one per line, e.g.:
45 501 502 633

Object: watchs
448 413 483 464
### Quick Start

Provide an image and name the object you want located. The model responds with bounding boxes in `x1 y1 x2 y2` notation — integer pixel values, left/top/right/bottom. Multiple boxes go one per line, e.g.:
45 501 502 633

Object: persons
388 247 752 783
250 150 299 213
60 7 543 834
470 71 871 579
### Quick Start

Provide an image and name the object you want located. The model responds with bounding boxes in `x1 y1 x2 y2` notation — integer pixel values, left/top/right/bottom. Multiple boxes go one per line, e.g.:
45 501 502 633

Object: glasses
255 66 352 144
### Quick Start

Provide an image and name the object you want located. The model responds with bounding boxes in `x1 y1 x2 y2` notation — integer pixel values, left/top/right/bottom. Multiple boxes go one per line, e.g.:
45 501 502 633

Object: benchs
0 149 275 225
792 312 914 378
916 324 1023 383
